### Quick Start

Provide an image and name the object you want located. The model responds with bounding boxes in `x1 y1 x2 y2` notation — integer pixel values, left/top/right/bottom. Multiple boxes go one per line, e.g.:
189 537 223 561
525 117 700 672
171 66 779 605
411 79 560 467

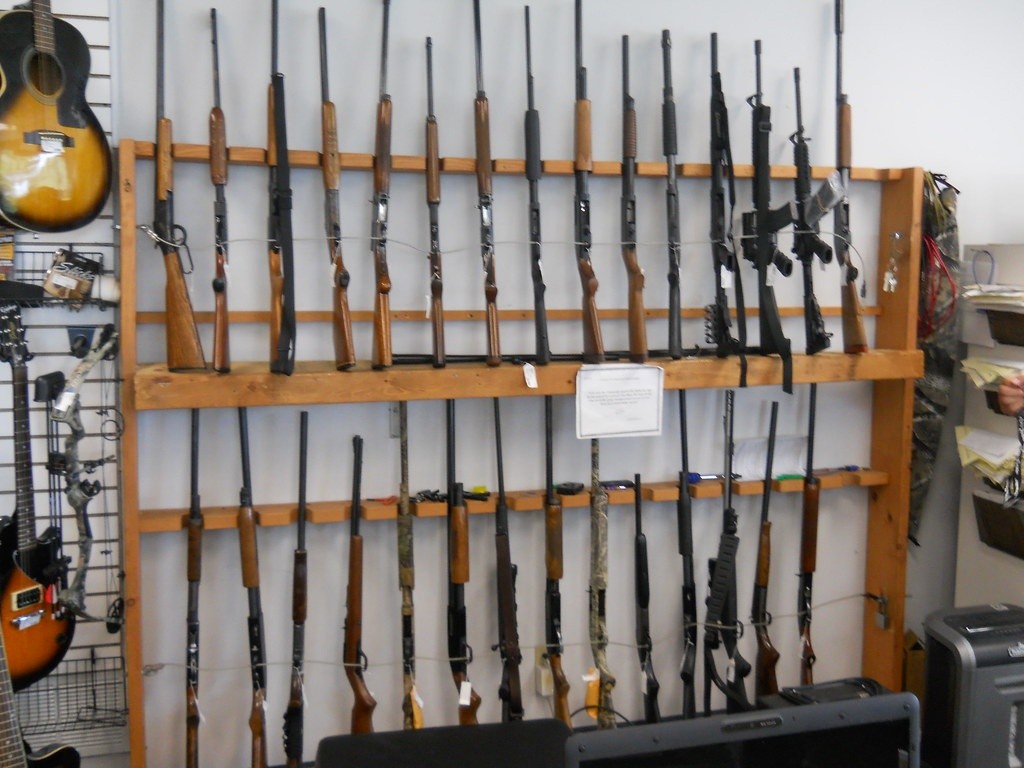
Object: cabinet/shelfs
117 136 924 768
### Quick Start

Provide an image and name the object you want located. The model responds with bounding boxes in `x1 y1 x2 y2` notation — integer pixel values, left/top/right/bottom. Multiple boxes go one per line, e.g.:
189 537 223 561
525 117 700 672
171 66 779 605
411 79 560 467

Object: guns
150 0 868 768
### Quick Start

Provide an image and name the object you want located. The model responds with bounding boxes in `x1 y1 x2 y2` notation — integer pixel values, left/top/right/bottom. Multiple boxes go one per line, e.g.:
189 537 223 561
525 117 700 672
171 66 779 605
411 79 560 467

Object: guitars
0 303 83 768
0 0 114 233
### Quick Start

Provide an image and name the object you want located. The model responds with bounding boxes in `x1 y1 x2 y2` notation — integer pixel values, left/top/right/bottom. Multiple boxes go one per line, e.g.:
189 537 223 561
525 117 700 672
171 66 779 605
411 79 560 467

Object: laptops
565 692 921 768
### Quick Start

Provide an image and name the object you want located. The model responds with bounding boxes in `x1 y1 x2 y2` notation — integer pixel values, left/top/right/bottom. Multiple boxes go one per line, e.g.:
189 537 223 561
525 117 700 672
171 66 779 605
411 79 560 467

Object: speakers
923 602 1024 768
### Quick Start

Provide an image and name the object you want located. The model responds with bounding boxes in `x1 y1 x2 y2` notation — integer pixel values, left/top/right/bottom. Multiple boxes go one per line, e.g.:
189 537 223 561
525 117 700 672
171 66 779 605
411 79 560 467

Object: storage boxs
903 628 925 721
